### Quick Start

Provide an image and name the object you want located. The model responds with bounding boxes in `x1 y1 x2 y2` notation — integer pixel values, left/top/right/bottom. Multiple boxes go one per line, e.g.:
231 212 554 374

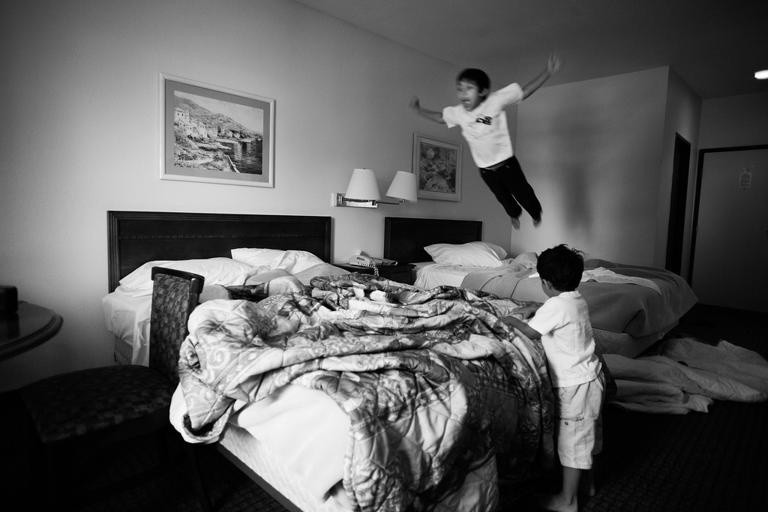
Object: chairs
22 266 211 512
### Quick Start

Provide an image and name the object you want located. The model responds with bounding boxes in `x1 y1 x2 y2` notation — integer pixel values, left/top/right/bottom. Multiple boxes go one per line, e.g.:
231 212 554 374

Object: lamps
334 169 418 210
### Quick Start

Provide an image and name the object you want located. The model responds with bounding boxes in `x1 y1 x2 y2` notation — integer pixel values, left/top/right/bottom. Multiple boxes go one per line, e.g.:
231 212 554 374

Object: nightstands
334 262 416 285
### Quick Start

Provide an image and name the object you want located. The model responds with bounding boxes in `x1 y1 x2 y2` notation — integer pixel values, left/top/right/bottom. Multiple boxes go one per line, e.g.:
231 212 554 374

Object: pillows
231 248 322 274
424 241 500 267
487 242 507 260
121 257 252 293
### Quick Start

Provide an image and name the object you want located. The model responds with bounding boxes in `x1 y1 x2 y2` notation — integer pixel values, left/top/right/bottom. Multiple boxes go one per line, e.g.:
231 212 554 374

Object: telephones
351 255 383 267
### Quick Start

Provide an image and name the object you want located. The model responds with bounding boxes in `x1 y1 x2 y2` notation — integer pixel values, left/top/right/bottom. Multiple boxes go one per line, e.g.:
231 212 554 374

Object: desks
0 301 63 360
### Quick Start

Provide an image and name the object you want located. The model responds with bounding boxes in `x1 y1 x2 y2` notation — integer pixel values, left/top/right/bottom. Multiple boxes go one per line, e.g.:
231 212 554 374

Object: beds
383 215 700 360
106 210 617 512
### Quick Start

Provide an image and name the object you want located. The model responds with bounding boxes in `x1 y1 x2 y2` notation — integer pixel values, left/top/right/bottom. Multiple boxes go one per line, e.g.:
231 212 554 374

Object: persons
499 242 609 512
411 50 563 230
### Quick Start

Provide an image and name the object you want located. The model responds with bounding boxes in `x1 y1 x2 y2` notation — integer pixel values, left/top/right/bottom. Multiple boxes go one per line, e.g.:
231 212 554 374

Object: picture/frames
412 133 463 201
159 75 276 190
